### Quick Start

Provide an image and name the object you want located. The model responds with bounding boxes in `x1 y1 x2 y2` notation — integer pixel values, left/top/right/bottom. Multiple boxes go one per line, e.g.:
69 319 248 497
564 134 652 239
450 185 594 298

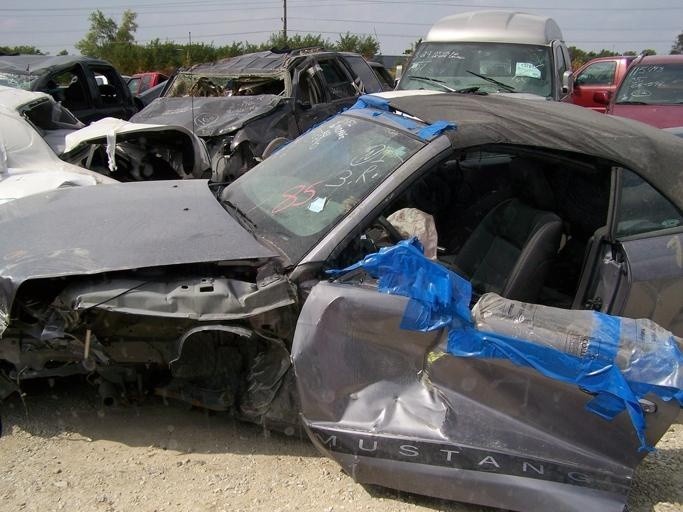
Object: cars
0 48 683 511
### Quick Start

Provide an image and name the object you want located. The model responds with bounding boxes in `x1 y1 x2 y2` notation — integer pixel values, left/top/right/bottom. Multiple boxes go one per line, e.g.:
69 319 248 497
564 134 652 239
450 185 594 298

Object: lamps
438 199 564 306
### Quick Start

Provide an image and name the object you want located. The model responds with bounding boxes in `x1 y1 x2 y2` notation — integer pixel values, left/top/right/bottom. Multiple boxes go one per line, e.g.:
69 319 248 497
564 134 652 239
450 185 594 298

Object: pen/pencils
393 9 574 104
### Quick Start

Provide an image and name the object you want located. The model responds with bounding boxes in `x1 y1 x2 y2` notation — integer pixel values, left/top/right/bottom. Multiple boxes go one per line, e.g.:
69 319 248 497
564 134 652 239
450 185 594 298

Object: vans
393 9 574 104
